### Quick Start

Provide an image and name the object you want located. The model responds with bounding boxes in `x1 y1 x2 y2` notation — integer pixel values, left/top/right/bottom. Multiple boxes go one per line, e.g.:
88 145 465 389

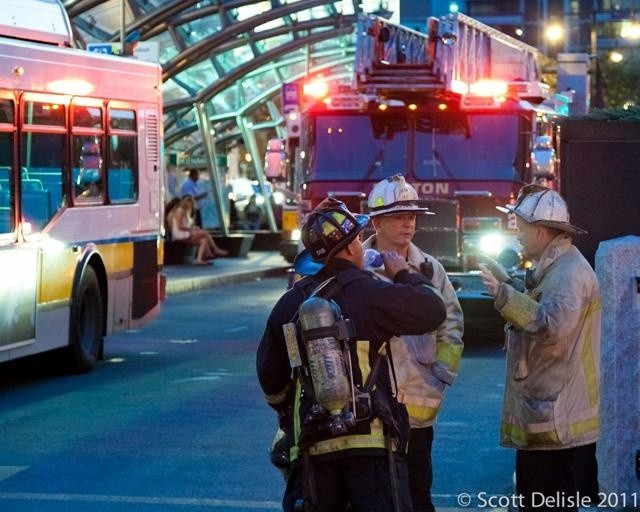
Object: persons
474 183 605 510
166 196 216 259
356 170 466 512
170 194 229 267
252 192 447 510
180 166 211 229
240 195 266 231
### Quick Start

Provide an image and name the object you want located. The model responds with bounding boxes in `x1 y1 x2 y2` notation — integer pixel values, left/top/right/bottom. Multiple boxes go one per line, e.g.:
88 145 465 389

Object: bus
0 22 166 375
280 113 301 262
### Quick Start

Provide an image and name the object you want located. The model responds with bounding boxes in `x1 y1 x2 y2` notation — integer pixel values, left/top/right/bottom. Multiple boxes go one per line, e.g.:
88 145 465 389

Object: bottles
330 411 357 434
364 249 386 271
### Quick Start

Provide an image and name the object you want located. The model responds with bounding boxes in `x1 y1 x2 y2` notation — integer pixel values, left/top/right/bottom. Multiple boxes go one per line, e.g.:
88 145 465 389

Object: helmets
505 183 588 238
365 173 435 216
293 195 372 278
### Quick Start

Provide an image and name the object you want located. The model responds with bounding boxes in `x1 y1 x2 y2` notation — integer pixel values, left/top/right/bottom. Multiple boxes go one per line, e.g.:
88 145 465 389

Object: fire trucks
264 6 568 342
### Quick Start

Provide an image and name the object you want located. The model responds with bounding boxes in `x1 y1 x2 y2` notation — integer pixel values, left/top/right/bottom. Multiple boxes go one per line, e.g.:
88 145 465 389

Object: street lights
545 12 639 64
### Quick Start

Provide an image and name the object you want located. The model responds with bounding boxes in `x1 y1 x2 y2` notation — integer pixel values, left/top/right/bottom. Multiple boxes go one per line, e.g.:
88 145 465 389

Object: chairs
0 165 134 236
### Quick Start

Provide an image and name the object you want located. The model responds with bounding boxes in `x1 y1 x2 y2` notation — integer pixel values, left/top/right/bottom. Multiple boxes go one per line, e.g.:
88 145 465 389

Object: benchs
164 228 280 267
